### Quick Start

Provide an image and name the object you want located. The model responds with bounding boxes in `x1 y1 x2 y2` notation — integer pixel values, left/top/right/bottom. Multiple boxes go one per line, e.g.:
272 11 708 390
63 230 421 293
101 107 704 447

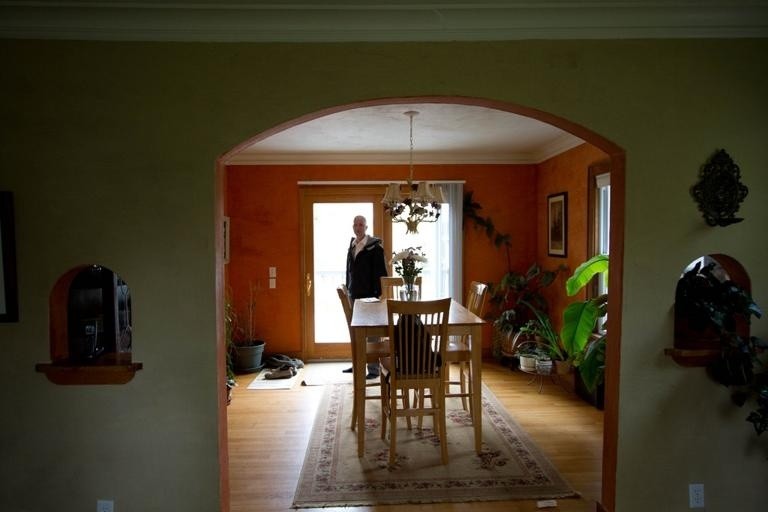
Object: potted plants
230 276 268 375
463 192 611 412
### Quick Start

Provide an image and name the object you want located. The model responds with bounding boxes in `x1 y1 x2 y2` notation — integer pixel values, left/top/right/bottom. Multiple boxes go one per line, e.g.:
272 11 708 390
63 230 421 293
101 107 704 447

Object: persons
342 216 388 380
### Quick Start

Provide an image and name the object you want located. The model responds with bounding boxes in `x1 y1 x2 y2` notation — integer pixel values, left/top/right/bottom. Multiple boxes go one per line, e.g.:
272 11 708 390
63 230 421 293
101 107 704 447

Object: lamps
379 111 448 235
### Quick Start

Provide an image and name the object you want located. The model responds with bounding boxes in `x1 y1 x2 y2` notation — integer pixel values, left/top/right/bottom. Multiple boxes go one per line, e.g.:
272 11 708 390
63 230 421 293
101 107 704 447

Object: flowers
388 246 429 300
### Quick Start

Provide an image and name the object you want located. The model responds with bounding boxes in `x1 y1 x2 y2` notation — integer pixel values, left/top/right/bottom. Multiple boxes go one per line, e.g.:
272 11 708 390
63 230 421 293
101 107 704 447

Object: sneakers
264 365 298 378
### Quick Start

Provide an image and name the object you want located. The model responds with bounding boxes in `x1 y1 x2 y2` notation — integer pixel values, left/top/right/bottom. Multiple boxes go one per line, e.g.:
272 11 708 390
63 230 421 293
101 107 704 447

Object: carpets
291 382 583 509
247 368 302 389
301 365 381 386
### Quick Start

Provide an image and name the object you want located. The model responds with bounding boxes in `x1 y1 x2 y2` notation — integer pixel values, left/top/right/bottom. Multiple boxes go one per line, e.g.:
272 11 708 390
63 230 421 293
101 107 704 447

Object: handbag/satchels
394 314 442 374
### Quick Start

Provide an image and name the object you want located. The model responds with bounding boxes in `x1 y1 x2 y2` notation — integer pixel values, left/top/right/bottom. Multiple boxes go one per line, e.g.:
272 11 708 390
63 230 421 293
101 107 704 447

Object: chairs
338 277 488 469
